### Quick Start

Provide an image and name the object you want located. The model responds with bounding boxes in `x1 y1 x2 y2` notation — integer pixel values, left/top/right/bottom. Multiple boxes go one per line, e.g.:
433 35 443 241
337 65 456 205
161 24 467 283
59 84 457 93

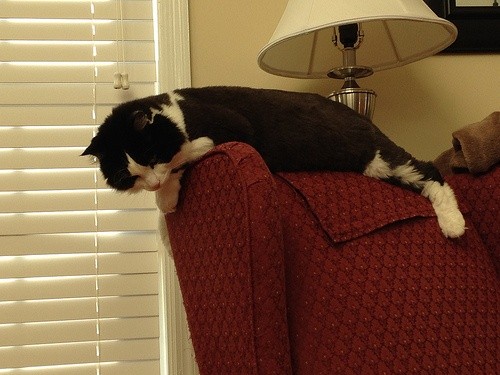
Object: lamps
257 0 457 122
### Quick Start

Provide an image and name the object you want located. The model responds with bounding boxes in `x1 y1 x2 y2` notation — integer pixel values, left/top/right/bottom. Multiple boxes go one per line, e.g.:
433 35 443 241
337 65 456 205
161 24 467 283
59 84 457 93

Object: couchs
165 142 500 375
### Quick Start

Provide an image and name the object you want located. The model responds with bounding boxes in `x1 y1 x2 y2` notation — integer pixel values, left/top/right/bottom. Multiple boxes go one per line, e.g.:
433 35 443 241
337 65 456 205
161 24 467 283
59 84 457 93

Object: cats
78 86 469 239
